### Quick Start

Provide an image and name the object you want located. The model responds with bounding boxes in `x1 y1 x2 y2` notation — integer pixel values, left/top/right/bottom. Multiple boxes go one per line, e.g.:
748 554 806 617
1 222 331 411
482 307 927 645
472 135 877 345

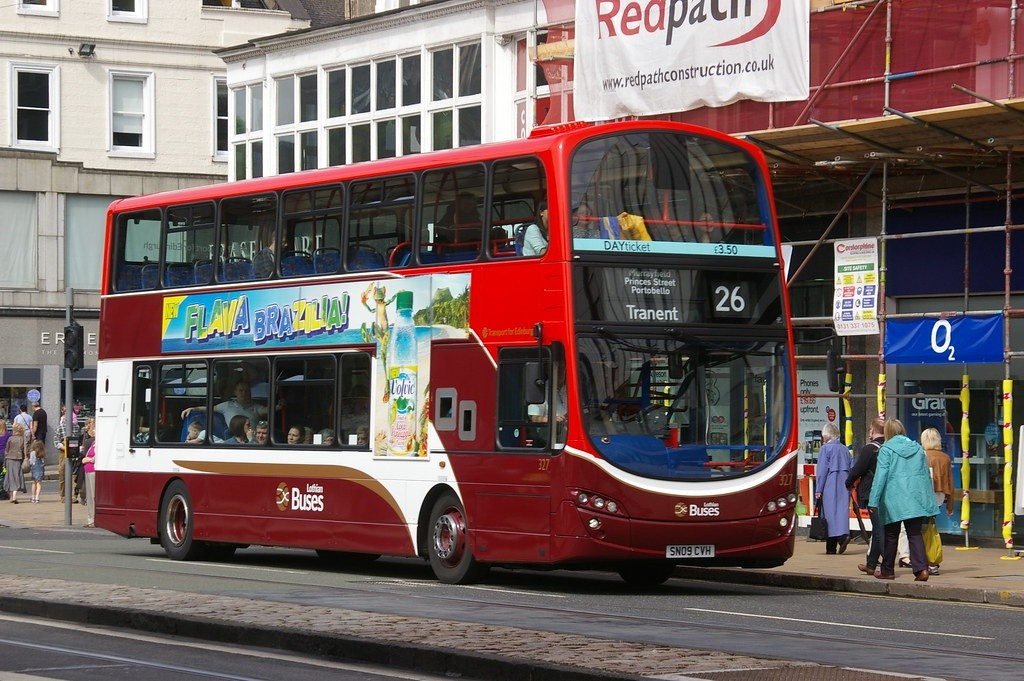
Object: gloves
845 480 853 489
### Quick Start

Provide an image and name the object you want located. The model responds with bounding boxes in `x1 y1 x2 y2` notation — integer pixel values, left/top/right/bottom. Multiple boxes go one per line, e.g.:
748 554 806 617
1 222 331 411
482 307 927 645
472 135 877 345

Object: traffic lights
63 325 85 371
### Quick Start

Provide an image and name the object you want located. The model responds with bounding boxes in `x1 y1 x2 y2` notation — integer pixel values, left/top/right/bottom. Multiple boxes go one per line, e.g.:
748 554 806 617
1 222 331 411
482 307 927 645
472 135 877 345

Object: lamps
78 43 96 56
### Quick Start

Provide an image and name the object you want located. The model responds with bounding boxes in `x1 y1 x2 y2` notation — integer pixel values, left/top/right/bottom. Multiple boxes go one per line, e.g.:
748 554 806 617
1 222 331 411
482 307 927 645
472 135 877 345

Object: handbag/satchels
21 458 30 473
923 450 934 493
920 514 942 566
810 496 827 540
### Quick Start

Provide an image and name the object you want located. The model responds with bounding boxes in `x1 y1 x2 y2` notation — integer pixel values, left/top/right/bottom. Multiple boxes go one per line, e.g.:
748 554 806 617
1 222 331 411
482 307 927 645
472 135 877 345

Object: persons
287 424 305 445
436 190 484 250
667 210 722 243
181 374 285 439
868 416 941 581
254 419 269 445
319 428 336 445
844 418 886 575
136 408 164 441
354 424 370 445
329 358 370 444
56 405 95 530
568 199 601 238
522 200 549 255
303 425 316 445
253 220 289 278
0 398 47 505
223 415 257 444
529 359 591 422
185 420 224 444
816 424 854 555
919 428 955 575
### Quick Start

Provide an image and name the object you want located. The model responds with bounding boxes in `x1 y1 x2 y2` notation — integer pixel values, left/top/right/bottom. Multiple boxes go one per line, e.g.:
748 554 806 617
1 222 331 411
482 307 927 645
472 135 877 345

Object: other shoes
839 536 850 554
30 497 39 504
928 565 939 575
83 522 95 528
11 499 18 504
858 564 874 575
874 571 894 580
915 570 929 581
61 496 87 506
826 551 836 554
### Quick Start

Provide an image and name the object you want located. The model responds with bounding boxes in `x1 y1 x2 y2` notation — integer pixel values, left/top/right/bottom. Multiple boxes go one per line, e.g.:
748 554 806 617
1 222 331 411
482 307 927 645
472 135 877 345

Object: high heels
866 555 880 564
899 557 912 568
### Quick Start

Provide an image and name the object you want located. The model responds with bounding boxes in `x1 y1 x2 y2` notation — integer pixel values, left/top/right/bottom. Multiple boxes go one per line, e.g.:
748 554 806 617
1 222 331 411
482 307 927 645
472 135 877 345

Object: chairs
118 223 531 291
181 413 226 442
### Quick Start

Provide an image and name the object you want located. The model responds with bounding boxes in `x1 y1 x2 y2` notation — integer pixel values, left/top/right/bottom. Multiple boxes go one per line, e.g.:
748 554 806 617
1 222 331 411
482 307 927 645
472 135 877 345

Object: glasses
246 423 250 428
259 421 267 425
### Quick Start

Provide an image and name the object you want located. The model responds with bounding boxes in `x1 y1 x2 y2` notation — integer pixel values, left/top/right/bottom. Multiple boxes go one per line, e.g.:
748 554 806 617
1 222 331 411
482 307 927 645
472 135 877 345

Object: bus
91 118 850 590
